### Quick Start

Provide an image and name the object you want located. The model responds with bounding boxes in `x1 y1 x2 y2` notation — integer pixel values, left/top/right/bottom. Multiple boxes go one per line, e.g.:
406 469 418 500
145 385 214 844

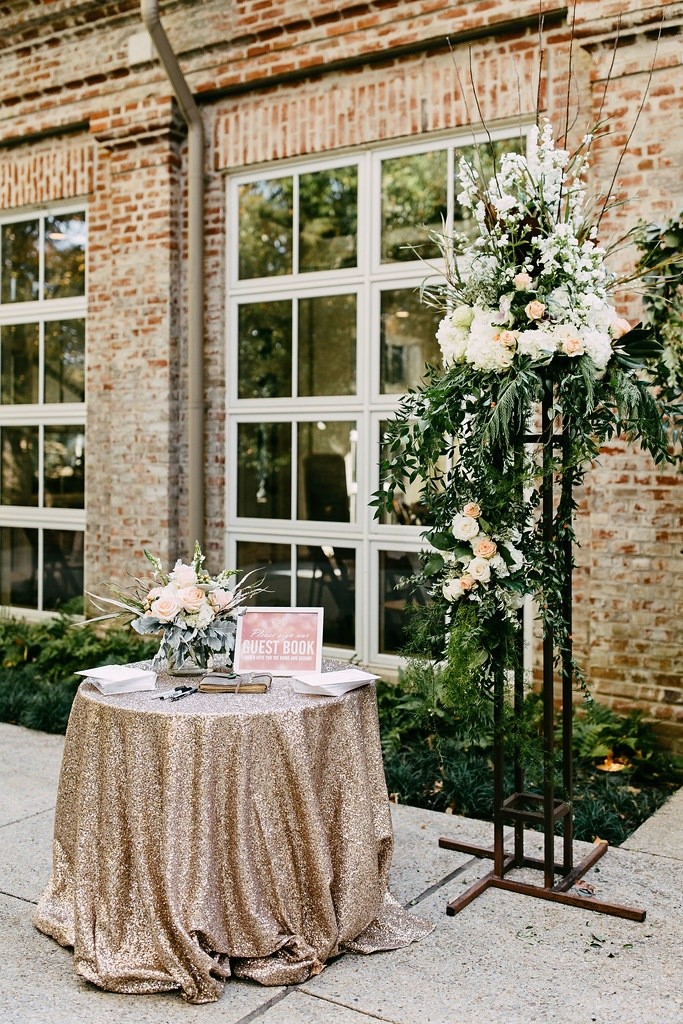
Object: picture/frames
233 606 324 678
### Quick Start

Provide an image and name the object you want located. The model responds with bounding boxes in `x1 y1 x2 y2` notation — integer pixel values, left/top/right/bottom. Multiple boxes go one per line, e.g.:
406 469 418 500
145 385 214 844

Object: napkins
74 664 157 697
291 668 383 698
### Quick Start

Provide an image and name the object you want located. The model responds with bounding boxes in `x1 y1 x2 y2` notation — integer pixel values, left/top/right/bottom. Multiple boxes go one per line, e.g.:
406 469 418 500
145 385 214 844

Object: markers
150 685 198 702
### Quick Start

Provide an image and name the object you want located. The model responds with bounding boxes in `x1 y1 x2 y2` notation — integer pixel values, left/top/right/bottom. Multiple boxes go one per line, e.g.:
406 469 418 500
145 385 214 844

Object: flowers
68 539 275 670
423 118 639 651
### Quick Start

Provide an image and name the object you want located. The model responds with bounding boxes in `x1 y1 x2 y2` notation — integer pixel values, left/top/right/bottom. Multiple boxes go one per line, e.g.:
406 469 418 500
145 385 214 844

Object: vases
166 632 208 677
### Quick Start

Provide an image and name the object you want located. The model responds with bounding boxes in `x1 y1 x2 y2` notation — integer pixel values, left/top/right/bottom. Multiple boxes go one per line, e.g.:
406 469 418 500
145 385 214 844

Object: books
199 670 273 695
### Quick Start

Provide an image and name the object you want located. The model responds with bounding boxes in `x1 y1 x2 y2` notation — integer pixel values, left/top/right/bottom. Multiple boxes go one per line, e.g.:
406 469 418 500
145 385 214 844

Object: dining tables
32 658 438 1005
